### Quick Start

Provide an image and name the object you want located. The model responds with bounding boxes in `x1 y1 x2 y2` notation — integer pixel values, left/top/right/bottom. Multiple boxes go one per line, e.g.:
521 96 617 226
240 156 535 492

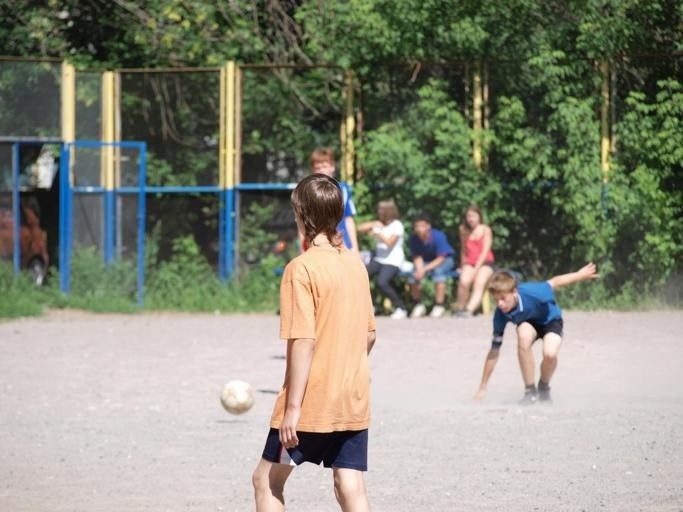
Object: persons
356 196 409 319
252 172 375 512
473 261 601 407
406 214 458 319
451 204 494 318
296 146 359 253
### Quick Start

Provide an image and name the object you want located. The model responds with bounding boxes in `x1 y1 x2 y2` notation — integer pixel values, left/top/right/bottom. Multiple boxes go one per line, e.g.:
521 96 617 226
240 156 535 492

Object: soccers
220 379 255 414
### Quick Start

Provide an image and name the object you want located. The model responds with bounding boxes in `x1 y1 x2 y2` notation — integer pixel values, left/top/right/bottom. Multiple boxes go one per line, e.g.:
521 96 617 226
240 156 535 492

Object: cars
0 192 50 287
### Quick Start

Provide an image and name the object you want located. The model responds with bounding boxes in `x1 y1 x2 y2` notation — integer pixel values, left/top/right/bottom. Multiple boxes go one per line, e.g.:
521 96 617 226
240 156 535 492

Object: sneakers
518 385 552 406
391 304 475 318
